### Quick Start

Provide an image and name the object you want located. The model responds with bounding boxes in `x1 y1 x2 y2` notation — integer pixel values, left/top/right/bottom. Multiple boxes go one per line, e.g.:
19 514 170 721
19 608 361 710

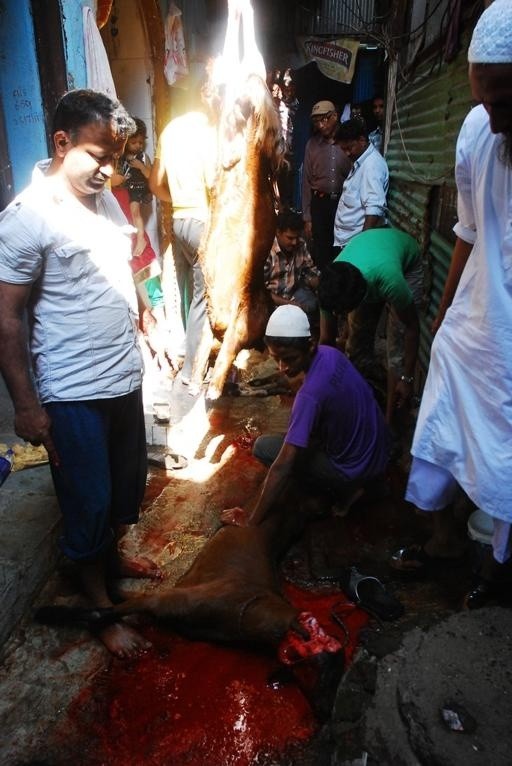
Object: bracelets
399 375 414 384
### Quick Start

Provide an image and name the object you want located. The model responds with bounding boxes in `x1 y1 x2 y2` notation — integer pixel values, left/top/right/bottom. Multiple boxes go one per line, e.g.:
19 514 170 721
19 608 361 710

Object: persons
116 115 153 260
219 303 392 530
366 95 385 157
106 166 180 373
263 209 322 347
387 0 512 607
331 115 392 352
1 86 164 665
301 96 353 250
146 76 214 389
313 225 425 413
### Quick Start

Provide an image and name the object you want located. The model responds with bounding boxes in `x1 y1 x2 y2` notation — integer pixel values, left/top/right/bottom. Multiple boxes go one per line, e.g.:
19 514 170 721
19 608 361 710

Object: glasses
311 113 332 122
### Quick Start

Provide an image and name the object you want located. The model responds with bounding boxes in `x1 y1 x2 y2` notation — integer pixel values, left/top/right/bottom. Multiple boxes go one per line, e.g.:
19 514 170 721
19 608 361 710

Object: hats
266 304 312 337
468 0 512 63
311 100 335 116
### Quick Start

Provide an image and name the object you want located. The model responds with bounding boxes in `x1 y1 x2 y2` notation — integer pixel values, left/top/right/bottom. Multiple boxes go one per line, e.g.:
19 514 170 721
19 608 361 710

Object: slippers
339 566 404 621
388 536 428 573
467 568 510 606
